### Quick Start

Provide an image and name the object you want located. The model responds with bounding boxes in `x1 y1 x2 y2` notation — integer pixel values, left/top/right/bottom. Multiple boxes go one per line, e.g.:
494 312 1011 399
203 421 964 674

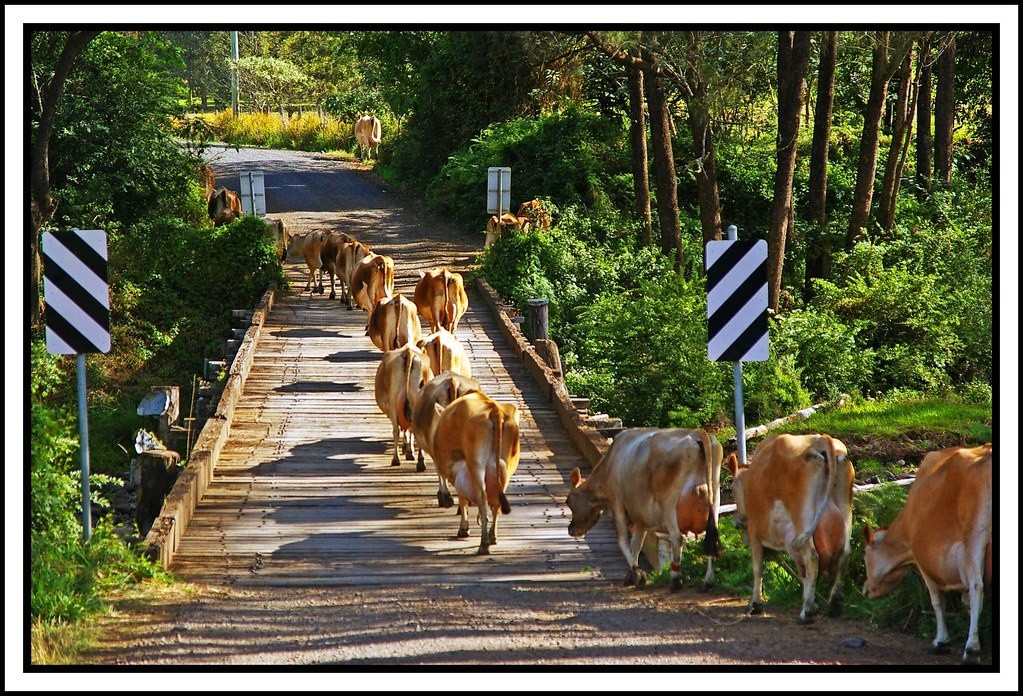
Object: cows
565 426 992 664
485 199 550 251
261 216 521 557
201 168 242 232
355 114 381 160
